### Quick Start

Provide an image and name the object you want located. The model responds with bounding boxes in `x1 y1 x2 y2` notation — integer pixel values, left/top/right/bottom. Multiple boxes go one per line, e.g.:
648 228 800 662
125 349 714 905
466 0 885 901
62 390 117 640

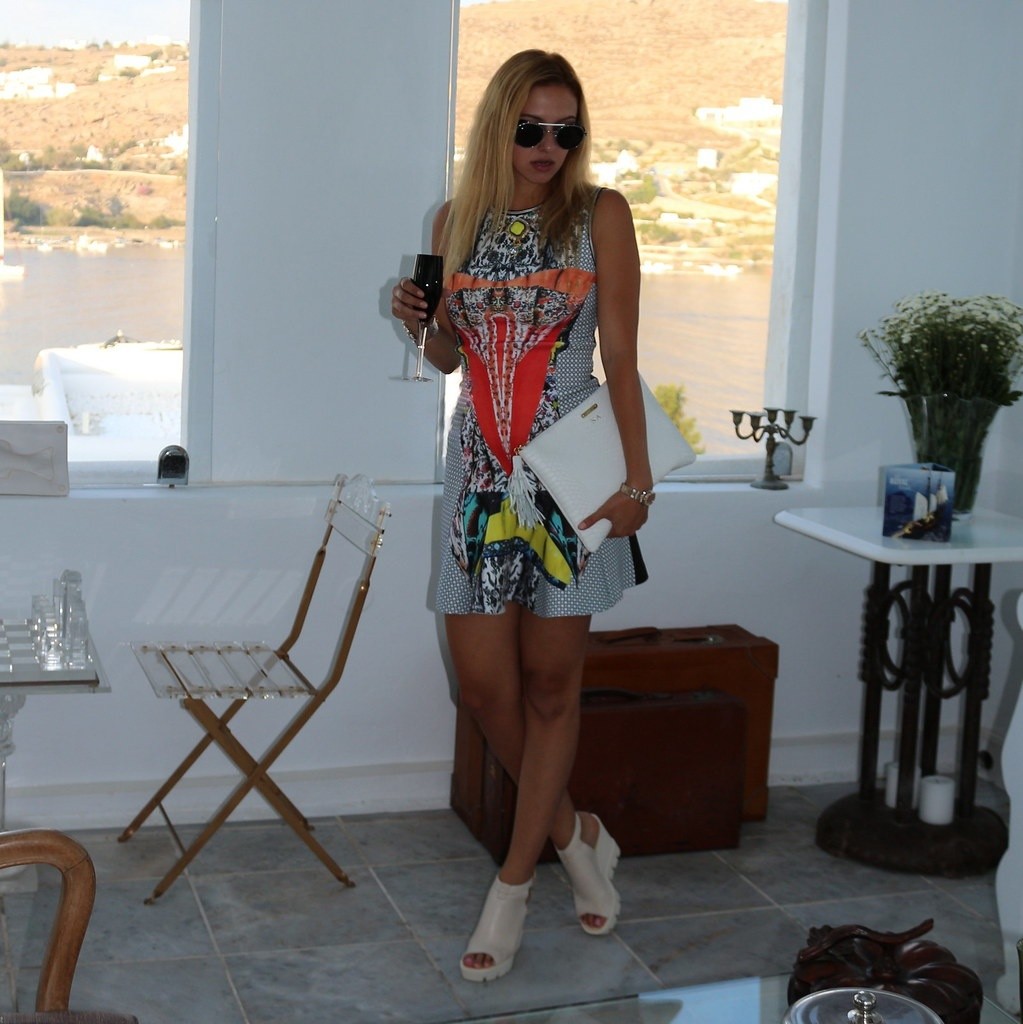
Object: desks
774 507 1023 879
0 589 112 880
451 971 1020 1024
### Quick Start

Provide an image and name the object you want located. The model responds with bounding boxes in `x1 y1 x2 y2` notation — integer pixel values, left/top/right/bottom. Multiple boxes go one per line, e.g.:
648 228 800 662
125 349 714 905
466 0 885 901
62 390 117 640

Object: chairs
0 828 138 1024
116 475 395 907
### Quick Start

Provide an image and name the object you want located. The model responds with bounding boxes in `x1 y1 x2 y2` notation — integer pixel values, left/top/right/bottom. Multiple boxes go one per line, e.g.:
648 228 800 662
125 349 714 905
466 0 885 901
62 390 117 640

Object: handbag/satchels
506 371 695 552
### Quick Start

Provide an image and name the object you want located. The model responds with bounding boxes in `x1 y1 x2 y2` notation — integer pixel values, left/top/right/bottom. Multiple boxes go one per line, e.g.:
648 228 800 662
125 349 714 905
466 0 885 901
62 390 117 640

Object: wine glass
397 254 444 384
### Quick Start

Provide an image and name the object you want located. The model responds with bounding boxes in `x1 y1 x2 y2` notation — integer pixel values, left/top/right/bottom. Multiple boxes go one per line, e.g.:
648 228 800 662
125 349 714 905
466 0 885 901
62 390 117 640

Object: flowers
856 286 1023 397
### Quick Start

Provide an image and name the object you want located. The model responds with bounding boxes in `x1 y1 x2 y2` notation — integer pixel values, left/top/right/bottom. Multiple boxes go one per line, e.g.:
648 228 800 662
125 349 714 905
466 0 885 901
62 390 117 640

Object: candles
885 760 921 808
919 774 954 825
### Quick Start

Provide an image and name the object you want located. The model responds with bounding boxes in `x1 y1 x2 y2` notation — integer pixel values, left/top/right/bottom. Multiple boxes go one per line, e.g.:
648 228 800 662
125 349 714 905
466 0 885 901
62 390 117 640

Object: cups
884 762 921 810
919 776 957 824
29 570 89 671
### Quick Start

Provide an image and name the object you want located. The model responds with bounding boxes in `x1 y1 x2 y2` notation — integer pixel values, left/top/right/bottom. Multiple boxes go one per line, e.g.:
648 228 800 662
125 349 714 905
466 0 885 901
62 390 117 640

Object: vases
901 393 999 522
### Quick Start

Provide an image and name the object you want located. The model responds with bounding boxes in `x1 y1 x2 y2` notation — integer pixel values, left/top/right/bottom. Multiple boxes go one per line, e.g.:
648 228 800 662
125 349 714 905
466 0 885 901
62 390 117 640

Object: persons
393 48 654 984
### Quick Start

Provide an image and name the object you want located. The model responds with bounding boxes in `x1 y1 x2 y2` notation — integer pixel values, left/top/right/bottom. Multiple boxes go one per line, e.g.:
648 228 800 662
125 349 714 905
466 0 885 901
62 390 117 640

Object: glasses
514 122 588 150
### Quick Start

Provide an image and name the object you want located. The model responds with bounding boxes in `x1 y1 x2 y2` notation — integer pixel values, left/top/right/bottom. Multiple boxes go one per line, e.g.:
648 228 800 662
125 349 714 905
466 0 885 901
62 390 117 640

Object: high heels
555 812 620 936
459 871 535 981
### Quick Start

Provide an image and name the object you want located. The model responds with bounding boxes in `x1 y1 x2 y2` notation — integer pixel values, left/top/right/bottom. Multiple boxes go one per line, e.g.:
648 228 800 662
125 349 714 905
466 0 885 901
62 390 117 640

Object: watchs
621 483 655 506
